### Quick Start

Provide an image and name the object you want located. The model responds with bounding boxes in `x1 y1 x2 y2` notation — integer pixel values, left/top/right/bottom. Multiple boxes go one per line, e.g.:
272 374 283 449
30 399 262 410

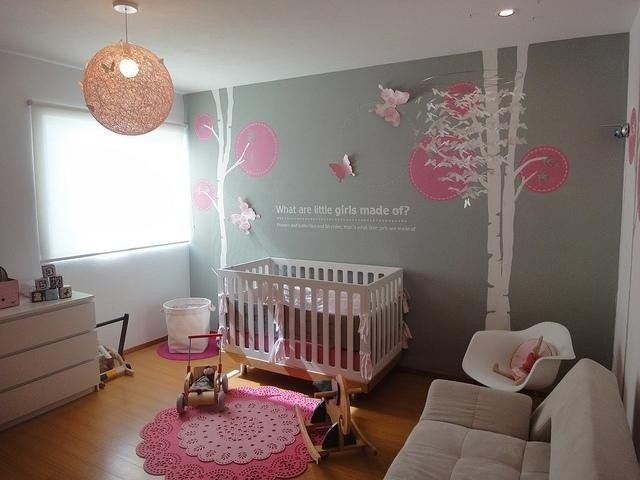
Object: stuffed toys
188 365 216 394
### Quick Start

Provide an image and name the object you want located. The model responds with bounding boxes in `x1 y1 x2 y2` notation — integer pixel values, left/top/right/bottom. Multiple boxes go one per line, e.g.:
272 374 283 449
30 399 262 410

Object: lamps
82 0 175 136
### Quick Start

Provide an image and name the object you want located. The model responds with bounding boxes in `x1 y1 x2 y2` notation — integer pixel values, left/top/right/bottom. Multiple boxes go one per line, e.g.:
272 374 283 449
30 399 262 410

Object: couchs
383 358 640 480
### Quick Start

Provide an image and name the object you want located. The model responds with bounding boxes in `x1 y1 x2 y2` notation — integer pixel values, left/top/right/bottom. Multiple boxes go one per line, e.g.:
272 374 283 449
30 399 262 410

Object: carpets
156 333 219 360
136 385 332 480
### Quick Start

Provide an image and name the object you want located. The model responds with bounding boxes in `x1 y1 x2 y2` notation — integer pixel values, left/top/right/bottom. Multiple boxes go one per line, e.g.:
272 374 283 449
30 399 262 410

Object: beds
217 256 403 402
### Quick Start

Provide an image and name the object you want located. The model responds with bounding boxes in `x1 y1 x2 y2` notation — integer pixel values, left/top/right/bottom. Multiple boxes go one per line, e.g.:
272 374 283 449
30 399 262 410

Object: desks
0 290 100 434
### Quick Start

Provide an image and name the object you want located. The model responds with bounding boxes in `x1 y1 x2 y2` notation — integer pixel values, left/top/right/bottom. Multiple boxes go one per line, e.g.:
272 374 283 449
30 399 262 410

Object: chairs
461 322 576 392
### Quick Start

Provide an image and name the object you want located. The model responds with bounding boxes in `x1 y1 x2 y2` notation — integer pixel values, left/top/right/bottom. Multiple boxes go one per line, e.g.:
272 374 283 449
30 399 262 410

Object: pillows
510 338 557 368
